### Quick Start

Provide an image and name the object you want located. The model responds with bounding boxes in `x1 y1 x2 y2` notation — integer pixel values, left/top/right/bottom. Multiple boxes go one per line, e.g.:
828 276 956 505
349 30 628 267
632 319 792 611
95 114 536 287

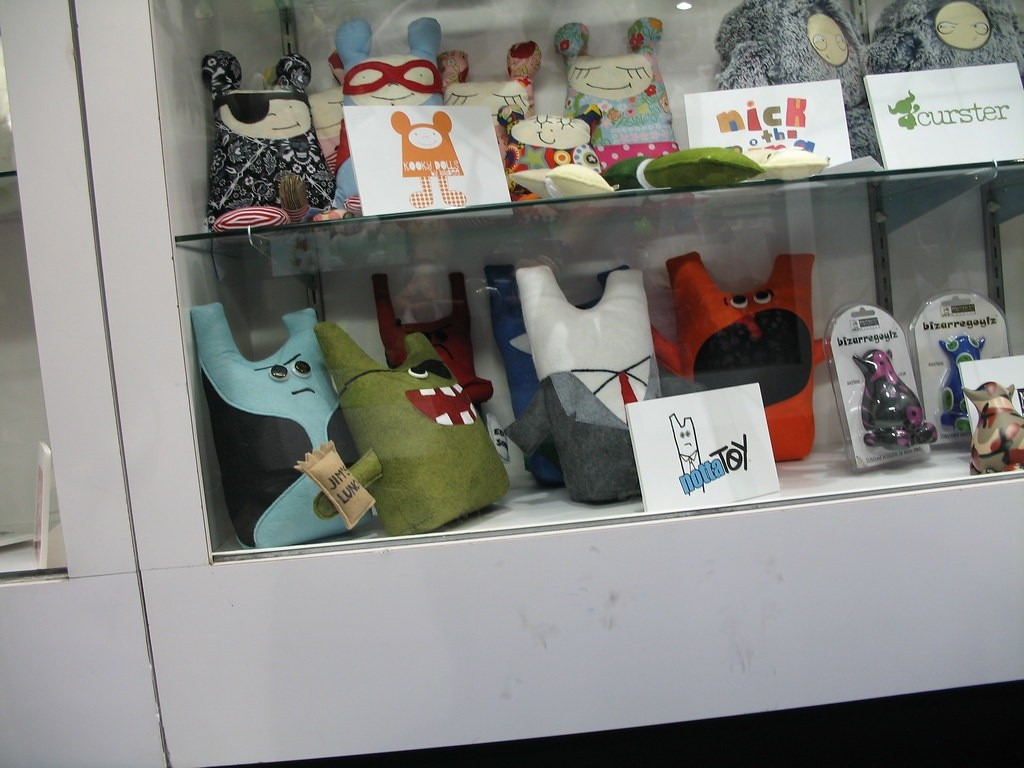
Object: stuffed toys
711 0 1024 167
189 252 833 548
199 15 678 227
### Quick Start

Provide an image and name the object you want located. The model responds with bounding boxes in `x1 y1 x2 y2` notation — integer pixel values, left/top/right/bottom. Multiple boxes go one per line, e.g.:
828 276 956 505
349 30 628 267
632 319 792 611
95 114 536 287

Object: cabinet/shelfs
1 0 1024 768
